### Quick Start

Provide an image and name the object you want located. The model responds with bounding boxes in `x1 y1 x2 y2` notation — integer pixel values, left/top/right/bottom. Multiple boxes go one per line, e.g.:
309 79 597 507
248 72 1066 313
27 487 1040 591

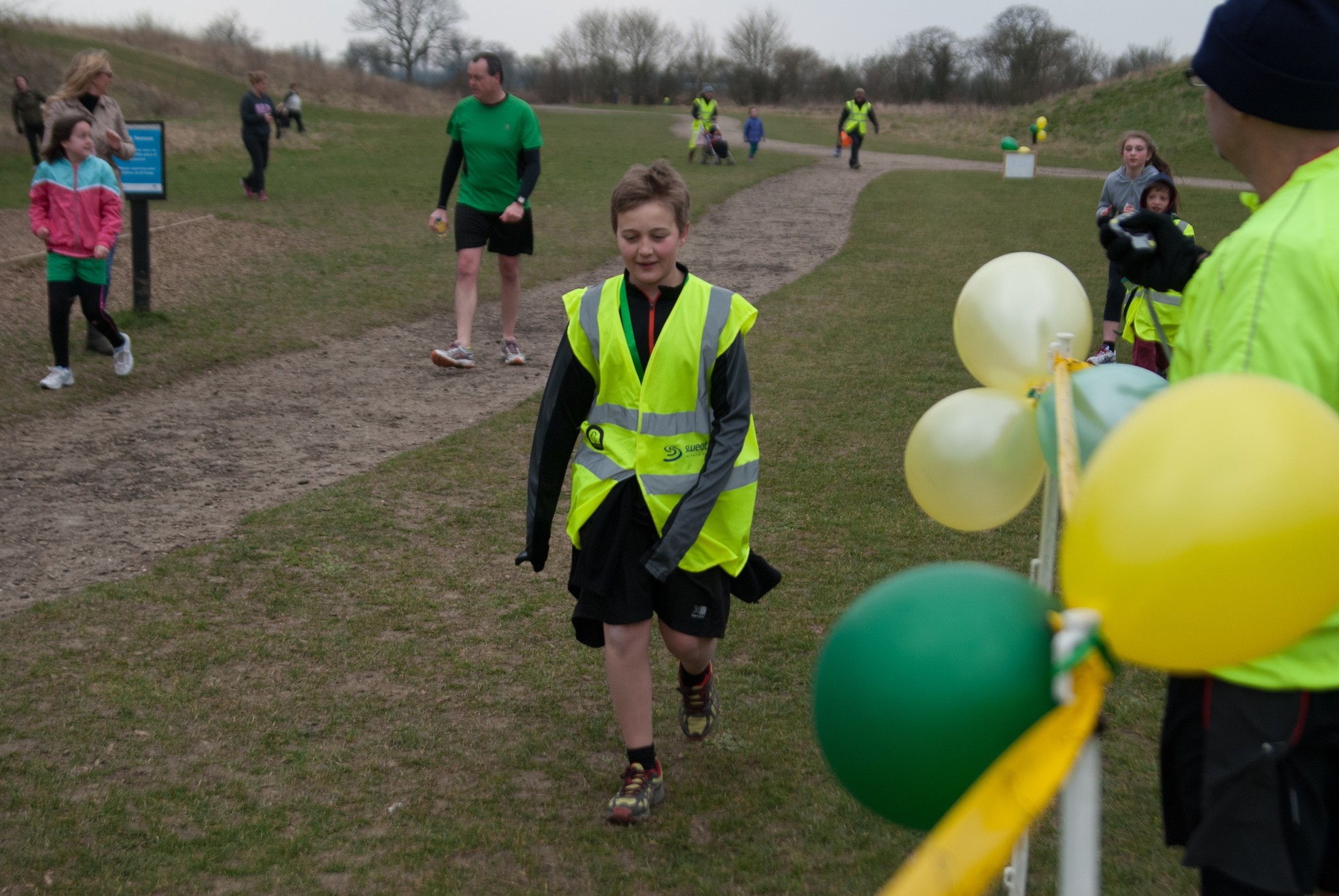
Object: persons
282 83 309 134
1096 0 1339 895
28 114 135 391
688 82 719 164
1088 129 1178 366
1120 172 1197 381
837 88 880 171
514 163 782 825
707 125 730 158
429 51 545 368
40 49 137 356
238 71 284 201
743 107 765 160
11 76 49 167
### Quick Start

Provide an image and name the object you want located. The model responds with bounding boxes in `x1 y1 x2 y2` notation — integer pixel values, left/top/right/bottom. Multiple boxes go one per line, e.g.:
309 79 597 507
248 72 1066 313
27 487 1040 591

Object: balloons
1058 372 1339 677
1036 116 1047 129
1000 136 1020 150
1017 146 1030 150
1036 363 1173 479
1036 129 1047 140
952 251 1094 397
904 386 1046 534
811 562 1068 833
1030 124 1038 132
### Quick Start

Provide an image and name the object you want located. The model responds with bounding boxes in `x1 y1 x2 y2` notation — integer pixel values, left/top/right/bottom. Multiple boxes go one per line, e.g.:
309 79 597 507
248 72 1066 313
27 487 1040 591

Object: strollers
698 115 737 165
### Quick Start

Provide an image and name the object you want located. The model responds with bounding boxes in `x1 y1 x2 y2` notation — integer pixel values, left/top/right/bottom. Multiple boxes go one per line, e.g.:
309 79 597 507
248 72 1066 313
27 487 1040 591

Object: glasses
1183 68 1210 87
100 70 114 78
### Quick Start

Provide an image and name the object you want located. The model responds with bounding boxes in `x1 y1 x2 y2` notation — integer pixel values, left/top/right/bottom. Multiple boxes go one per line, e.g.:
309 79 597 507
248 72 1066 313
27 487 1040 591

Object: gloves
1096 208 1211 293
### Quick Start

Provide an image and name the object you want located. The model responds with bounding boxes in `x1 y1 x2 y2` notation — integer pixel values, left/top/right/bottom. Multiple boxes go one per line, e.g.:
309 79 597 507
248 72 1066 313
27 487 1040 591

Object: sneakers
607 757 666 823
688 151 737 168
1086 343 1116 366
240 177 269 201
499 335 526 365
114 332 134 376
40 366 75 389
676 657 720 740
431 340 476 369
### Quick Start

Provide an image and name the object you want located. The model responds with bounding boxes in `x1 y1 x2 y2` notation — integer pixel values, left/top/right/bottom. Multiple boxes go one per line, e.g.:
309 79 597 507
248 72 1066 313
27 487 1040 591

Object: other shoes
747 156 755 162
848 156 862 169
833 145 842 157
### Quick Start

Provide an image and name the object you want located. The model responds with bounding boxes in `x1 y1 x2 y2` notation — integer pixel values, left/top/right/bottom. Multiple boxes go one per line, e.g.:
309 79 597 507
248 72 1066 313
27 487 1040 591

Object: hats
702 82 715 93
1190 1 1339 132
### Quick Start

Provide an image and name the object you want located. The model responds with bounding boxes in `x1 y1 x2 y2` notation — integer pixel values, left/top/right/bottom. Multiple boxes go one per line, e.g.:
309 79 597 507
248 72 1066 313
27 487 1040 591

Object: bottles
434 215 447 236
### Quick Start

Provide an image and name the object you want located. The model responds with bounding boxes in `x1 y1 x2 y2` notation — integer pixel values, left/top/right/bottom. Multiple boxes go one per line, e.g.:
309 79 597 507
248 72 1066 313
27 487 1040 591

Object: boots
85 323 114 356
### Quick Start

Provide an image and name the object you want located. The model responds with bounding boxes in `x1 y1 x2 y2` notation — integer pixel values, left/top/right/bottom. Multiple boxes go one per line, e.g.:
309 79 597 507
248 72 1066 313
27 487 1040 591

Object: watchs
515 195 525 205
